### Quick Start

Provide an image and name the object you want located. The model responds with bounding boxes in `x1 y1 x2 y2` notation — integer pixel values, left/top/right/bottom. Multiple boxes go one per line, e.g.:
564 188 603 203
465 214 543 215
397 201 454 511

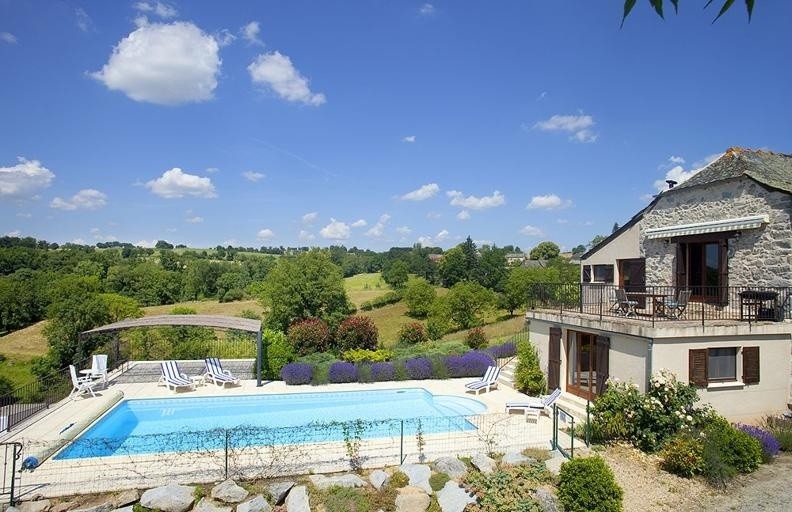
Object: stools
191 376 205 388
524 409 540 424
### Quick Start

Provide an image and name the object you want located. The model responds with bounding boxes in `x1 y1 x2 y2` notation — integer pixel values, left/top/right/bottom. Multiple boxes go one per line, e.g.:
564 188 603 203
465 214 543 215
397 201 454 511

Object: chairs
466 366 502 395
158 360 196 394
506 387 562 417
90 355 109 390
203 357 240 389
614 288 638 317
655 289 692 319
68 365 100 401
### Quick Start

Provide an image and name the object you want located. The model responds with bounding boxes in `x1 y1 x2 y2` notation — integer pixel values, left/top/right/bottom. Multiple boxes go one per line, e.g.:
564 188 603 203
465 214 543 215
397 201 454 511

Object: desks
79 369 104 395
625 291 675 318
